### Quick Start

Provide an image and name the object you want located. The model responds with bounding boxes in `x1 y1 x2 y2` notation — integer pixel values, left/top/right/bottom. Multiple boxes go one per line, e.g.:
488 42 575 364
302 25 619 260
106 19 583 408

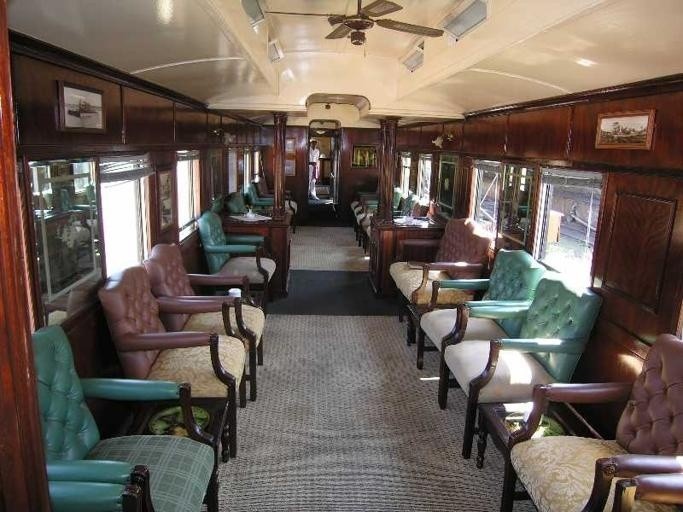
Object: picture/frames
594 108 656 150
57 79 105 135
436 154 458 210
350 145 377 170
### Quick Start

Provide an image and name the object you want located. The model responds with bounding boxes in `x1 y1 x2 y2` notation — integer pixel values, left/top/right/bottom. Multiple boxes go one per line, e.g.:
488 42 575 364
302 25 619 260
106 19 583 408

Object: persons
307 138 320 201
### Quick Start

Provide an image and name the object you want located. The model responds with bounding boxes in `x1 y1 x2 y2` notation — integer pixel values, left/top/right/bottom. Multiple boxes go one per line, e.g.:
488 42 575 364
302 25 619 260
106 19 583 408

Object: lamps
207 128 237 146
351 29 365 45
432 134 456 148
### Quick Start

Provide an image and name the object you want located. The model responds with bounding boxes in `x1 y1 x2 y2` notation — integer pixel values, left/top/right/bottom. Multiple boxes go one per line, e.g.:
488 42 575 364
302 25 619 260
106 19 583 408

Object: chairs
500 333 682 511
98 267 247 460
199 209 278 319
350 188 435 255
225 174 299 235
417 247 546 409
438 270 602 460
141 241 266 403
30 324 222 512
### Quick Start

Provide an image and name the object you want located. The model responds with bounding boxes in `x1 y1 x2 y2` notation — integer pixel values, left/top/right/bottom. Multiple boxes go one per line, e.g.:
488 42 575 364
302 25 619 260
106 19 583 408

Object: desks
120 396 228 503
477 398 592 511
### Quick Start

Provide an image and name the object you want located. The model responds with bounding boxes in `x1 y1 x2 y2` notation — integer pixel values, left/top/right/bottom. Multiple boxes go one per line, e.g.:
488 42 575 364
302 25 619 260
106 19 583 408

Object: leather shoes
308 194 319 200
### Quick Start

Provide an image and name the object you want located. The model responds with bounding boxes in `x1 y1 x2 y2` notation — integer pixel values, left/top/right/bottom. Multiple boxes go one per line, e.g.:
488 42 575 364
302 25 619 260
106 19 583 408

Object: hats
310 137 318 143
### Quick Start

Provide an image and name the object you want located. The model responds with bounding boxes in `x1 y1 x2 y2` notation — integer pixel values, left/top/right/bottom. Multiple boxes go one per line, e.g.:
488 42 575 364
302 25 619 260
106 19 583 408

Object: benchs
391 217 501 347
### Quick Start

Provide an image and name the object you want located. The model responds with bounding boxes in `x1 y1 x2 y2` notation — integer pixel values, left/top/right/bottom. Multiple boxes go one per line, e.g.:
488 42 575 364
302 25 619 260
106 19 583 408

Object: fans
265 0 445 38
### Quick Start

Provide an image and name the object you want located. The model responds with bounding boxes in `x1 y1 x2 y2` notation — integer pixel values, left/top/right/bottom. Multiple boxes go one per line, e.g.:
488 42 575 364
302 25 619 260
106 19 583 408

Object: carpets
289 226 371 271
197 314 536 512
